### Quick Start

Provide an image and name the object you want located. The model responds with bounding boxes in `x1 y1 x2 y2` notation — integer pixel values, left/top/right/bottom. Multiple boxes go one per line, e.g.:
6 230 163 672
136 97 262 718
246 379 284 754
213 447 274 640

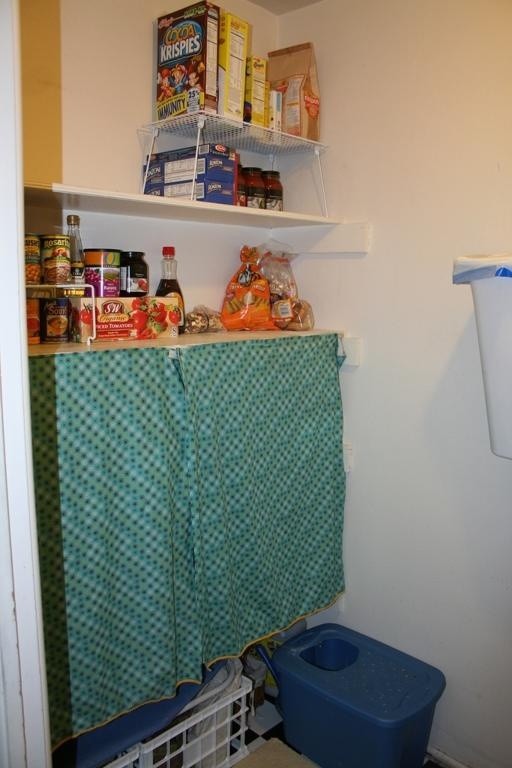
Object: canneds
27 298 41 345
41 299 68 343
40 233 72 284
262 171 283 211
236 164 246 206
83 248 120 267
25 234 40 285
245 167 265 209
83 266 120 296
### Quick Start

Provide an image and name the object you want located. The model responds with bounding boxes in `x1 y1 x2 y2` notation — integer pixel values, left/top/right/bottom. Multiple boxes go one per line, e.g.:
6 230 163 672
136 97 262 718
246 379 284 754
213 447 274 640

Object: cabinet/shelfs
25 182 342 358
140 110 329 218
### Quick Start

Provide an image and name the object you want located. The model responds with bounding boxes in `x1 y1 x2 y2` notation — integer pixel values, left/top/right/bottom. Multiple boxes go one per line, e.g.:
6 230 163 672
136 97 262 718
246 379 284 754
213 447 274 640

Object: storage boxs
271 623 446 768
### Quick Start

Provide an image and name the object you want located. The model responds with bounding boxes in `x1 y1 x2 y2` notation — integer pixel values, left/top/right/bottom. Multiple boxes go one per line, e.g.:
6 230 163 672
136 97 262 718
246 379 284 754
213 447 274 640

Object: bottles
65 214 86 296
155 246 185 334
